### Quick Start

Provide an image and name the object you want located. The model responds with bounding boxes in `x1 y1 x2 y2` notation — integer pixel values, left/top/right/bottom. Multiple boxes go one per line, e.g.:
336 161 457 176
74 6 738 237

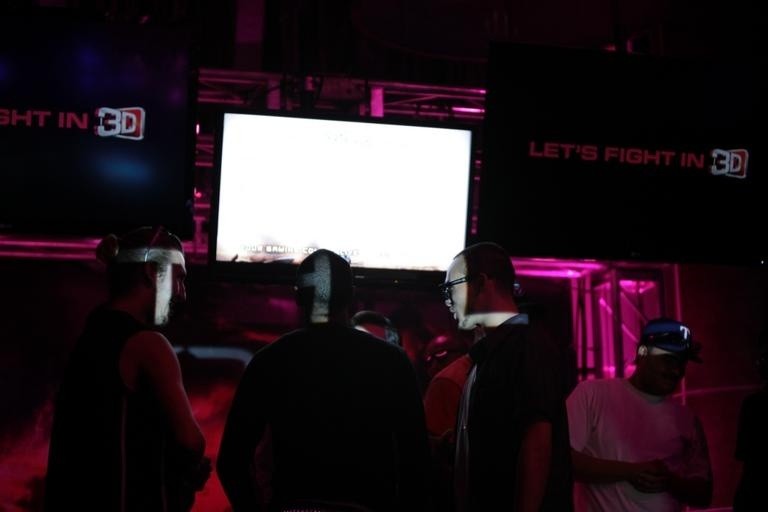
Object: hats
640 317 702 364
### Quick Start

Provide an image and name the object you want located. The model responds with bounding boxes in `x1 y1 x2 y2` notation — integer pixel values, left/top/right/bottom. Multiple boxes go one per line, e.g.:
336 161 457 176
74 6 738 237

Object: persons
43 225 212 512
214 248 433 511
436 241 577 510
565 317 714 511
351 309 399 347
729 340 767 512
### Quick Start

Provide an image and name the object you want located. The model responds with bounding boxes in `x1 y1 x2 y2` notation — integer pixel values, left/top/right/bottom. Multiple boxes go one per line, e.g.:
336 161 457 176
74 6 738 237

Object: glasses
437 275 470 300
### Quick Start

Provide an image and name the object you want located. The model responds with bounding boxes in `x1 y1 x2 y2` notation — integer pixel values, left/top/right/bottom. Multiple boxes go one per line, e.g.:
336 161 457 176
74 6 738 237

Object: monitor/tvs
209 107 476 292
478 39 768 265
0 30 197 233
